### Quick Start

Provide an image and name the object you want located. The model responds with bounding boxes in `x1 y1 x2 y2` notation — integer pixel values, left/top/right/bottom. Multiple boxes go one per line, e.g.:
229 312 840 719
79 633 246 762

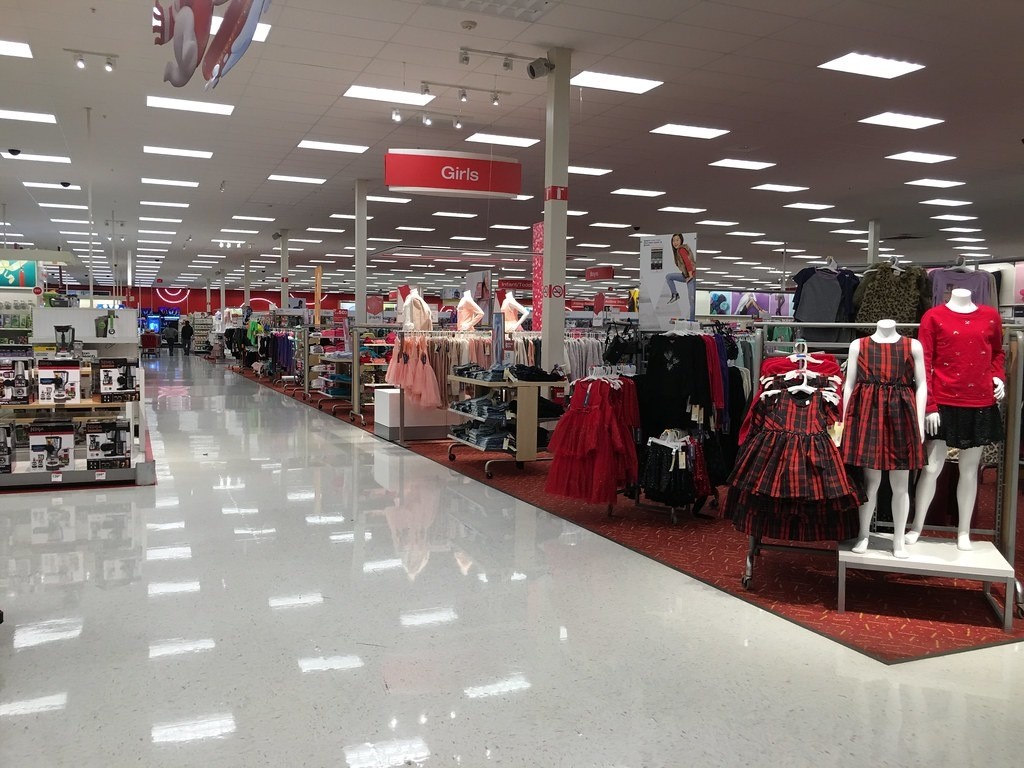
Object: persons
215 311 222 331
665 234 695 321
299 300 304 308
739 293 765 315
776 294 785 315
181 321 193 356
454 289 460 298
710 294 726 314
458 290 484 331
905 288 1006 551
403 290 433 330
629 288 639 312
501 292 529 331
224 308 232 327
163 323 178 356
201 313 205 316
843 319 927 558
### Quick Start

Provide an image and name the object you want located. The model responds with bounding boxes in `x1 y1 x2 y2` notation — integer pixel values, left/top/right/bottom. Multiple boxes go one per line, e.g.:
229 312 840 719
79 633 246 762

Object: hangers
862 257 926 278
395 331 604 346
930 258 995 279
659 318 757 338
752 340 845 406
570 364 651 391
646 430 710 450
797 256 858 281
272 329 301 337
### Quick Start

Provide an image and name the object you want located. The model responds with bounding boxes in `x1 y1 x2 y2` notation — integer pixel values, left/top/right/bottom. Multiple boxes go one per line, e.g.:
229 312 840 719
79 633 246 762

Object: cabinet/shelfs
177 307 399 358
349 327 502 427
447 376 570 475
1 247 156 488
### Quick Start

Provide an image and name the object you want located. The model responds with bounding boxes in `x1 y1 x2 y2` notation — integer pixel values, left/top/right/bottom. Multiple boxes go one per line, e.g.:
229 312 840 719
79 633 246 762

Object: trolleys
139 333 161 358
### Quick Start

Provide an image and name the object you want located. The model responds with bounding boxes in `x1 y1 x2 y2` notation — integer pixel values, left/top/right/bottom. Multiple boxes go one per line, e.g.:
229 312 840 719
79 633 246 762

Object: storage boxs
39 361 80 405
30 428 74 472
1 358 31 405
84 418 132 469
0 418 17 474
98 358 140 405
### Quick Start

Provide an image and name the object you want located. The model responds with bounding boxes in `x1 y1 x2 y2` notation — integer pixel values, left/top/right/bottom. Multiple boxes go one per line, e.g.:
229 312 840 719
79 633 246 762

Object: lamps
420 81 516 108
119 234 127 243
107 232 113 242
186 235 192 242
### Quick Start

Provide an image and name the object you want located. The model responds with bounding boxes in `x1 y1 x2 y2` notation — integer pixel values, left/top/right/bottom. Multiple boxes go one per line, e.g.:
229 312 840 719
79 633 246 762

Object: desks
838 540 1015 631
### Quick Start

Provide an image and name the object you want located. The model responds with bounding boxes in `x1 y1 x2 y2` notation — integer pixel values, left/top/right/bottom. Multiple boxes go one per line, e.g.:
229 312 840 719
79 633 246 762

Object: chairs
139 333 161 358
